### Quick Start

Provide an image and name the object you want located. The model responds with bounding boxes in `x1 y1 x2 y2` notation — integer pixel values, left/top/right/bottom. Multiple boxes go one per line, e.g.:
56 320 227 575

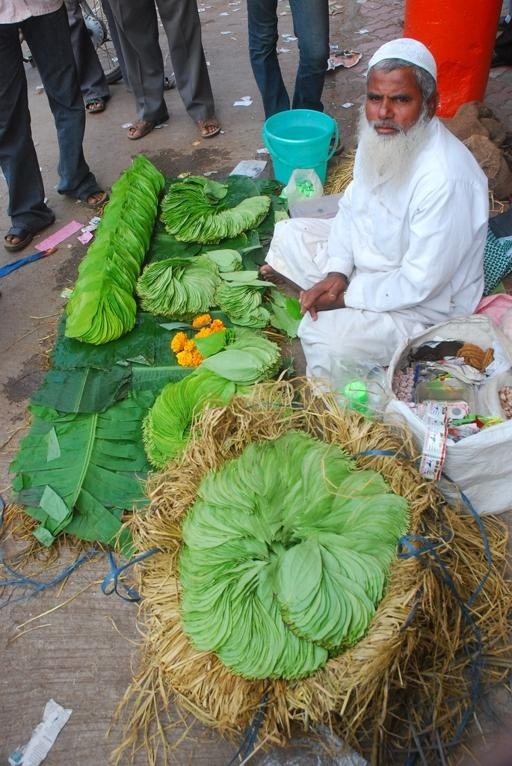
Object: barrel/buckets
404 0 503 119
261 109 340 188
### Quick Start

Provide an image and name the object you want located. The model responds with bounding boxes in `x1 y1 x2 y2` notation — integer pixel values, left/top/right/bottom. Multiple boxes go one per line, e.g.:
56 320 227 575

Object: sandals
3 225 34 252
79 184 109 209
127 110 170 140
85 97 106 115
327 134 345 157
196 114 221 137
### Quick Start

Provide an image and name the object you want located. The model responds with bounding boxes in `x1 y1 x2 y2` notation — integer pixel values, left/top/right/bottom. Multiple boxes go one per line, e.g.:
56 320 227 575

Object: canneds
344 380 371 419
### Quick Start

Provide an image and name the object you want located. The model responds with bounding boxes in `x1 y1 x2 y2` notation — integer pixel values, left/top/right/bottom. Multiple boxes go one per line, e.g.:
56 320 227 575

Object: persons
0 0 109 250
259 36 493 395
245 1 345 156
62 0 176 114
97 0 221 141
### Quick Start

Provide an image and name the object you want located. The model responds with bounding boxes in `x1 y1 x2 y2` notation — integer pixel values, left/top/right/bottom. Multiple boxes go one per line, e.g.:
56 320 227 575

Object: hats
367 37 436 81
